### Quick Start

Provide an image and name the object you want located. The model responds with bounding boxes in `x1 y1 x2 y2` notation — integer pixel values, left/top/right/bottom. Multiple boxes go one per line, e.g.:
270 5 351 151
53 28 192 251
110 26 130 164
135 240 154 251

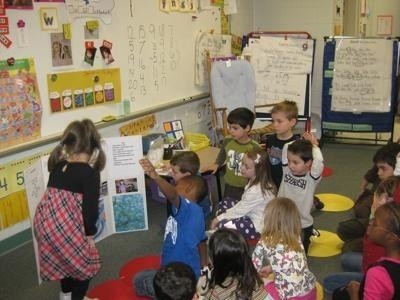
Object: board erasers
103 115 116 122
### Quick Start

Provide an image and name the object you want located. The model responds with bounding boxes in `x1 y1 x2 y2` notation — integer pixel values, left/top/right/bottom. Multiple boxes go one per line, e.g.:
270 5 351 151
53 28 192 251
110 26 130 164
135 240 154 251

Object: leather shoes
312 228 320 236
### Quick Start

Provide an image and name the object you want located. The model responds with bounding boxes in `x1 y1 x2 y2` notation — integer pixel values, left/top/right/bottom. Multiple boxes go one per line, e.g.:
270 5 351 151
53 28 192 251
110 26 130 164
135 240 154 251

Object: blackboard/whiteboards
0 0 232 158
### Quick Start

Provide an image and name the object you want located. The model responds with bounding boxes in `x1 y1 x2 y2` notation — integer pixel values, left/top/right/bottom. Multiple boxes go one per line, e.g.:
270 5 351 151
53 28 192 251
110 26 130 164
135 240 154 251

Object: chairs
207 53 280 149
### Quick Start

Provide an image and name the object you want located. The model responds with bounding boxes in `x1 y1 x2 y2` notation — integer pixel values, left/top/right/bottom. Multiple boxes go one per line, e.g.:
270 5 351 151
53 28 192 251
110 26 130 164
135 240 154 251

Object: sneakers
60 290 72 299
314 196 324 209
83 295 99 300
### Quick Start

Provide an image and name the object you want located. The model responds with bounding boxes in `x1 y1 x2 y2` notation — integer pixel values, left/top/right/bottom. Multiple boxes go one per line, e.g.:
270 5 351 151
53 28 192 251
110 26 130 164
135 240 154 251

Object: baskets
184 132 209 152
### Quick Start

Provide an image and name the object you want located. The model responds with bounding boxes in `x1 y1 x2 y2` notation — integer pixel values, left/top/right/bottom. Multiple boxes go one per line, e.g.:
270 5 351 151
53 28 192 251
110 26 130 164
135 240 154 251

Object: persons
152 261 200 300
101 47 114 64
274 132 324 256
119 180 132 193
332 201 400 300
197 227 275 300
266 100 320 193
132 158 208 300
85 48 95 64
252 198 317 300
200 108 264 203
322 140 399 293
170 151 210 221
34 118 106 300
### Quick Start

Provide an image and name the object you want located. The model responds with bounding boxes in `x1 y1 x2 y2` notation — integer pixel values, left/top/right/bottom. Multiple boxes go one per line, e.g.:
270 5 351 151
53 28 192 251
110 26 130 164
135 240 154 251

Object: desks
146 145 226 224
